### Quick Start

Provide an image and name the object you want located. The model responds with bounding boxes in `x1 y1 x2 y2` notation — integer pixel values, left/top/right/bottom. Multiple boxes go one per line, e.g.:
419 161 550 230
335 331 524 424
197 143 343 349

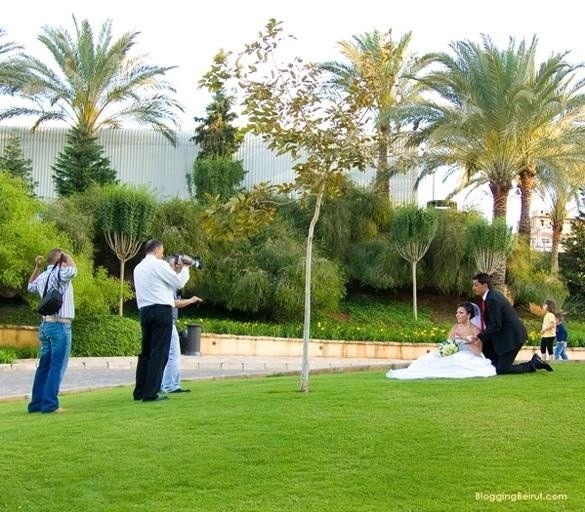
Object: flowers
437 340 464 358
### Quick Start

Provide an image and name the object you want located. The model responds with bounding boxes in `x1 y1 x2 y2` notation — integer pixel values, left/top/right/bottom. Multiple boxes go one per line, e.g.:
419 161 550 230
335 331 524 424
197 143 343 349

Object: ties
480 300 485 332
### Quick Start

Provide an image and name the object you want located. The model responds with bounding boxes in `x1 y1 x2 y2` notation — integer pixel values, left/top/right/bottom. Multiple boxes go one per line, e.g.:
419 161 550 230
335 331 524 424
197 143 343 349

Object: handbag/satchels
38 290 63 316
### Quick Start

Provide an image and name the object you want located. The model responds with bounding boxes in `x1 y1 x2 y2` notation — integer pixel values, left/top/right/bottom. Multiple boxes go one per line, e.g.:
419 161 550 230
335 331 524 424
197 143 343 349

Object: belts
43 317 71 322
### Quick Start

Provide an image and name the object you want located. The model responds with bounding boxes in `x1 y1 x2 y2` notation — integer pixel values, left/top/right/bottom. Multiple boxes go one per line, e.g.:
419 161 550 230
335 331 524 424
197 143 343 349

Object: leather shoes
532 354 553 371
54 407 67 412
171 387 191 392
156 395 168 401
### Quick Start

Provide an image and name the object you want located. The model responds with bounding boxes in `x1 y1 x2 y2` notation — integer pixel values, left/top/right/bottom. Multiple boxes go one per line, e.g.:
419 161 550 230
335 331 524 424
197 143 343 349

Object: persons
386 301 499 380
554 312 570 361
471 273 555 375
540 299 559 361
26 248 78 414
132 239 192 402
159 255 204 397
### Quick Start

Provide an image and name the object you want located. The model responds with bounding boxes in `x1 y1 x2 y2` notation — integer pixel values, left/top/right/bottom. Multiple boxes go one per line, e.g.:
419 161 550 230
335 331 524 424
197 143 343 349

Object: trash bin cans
180 324 202 355
